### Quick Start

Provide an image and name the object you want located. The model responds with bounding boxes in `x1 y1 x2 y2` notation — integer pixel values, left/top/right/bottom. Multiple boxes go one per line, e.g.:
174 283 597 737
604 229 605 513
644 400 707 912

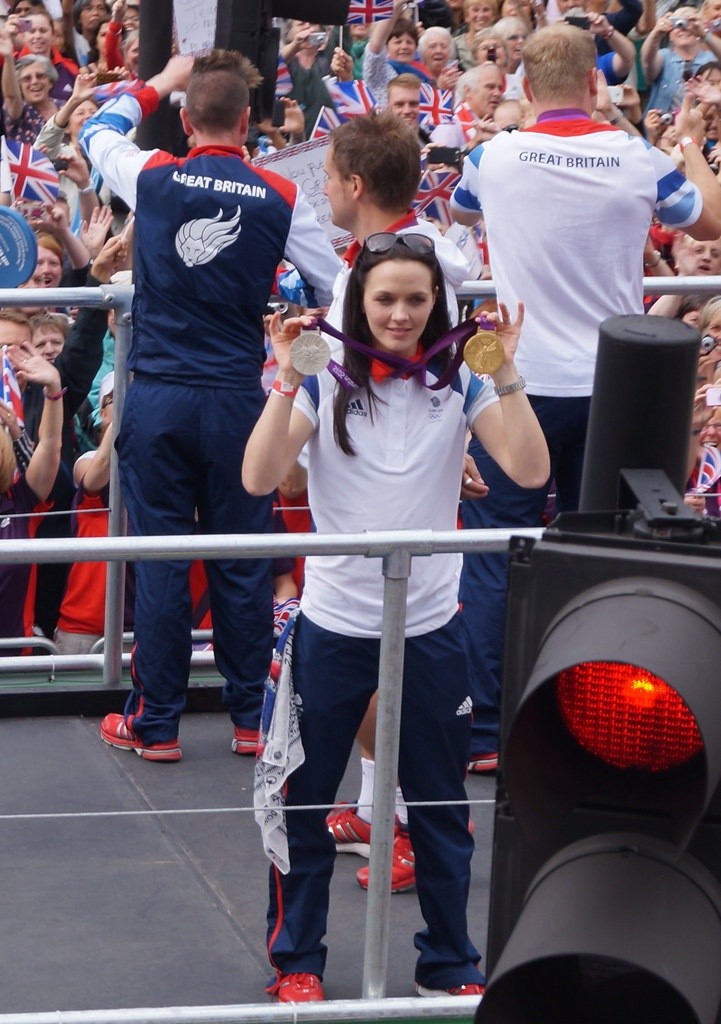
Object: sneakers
232 723 265 754
278 972 324 1002
324 802 401 859
415 978 485 997
355 819 477 892
101 712 182 761
464 752 500 773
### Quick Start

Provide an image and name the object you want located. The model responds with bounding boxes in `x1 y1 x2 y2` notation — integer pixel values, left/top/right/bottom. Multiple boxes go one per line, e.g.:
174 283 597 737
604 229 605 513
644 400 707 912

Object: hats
99 372 137 400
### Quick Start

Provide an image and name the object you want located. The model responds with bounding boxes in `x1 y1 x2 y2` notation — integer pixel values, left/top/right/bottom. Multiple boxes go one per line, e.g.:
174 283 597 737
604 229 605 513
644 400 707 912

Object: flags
345 0 394 25
276 56 294 97
93 80 128 104
698 446 721 493
327 79 379 123
419 82 452 126
413 169 463 226
0 351 24 430
0 135 60 205
310 106 350 139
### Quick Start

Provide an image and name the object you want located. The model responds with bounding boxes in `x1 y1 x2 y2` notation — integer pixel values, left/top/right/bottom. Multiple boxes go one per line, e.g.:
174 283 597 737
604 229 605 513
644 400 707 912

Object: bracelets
680 137 699 154
610 112 623 125
272 380 297 397
43 387 67 400
494 375 527 395
700 28 711 42
603 26 614 39
645 250 661 267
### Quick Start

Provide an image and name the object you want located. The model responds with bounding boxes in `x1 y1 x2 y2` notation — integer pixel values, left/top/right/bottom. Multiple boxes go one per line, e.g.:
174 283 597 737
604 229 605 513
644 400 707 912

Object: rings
7 413 10 417
464 478 472 485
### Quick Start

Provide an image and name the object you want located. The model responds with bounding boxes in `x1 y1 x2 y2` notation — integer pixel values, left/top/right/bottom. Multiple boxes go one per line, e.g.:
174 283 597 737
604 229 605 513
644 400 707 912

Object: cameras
706 387 721 406
49 159 68 170
266 295 303 321
658 112 675 126
97 73 122 87
17 19 32 32
308 32 327 46
700 334 718 355
668 17 687 29
272 100 285 127
708 17 721 32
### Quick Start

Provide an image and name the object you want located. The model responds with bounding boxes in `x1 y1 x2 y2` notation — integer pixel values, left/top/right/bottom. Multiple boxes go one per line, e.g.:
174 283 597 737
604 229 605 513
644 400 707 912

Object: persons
242 233 550 1000
322 109 488 892
0 0 721 655
452 22 721 775
79 47 341 758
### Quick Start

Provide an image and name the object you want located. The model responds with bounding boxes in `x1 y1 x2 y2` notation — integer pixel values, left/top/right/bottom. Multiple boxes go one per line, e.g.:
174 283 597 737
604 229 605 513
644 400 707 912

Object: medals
463 333 505 374
290 332 332 375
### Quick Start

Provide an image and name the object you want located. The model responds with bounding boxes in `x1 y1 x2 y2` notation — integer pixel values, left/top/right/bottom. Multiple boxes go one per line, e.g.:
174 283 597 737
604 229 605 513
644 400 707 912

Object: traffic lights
474 534 720 1024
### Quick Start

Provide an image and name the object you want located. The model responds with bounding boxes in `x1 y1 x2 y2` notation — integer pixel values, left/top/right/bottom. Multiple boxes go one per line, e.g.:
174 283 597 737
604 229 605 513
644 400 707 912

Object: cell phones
14 201 49 222
608 86 624 103
428 146 460 165
565 16 591 31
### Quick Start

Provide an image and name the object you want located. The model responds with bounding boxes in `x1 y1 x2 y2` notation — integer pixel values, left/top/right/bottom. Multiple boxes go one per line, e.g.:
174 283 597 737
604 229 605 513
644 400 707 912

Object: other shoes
188 642 212 651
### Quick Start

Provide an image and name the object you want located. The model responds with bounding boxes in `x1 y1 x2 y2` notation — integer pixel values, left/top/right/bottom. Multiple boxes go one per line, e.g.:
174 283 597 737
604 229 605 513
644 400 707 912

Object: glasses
22 74 48 85
364 232 437 253
683 60 693 82
